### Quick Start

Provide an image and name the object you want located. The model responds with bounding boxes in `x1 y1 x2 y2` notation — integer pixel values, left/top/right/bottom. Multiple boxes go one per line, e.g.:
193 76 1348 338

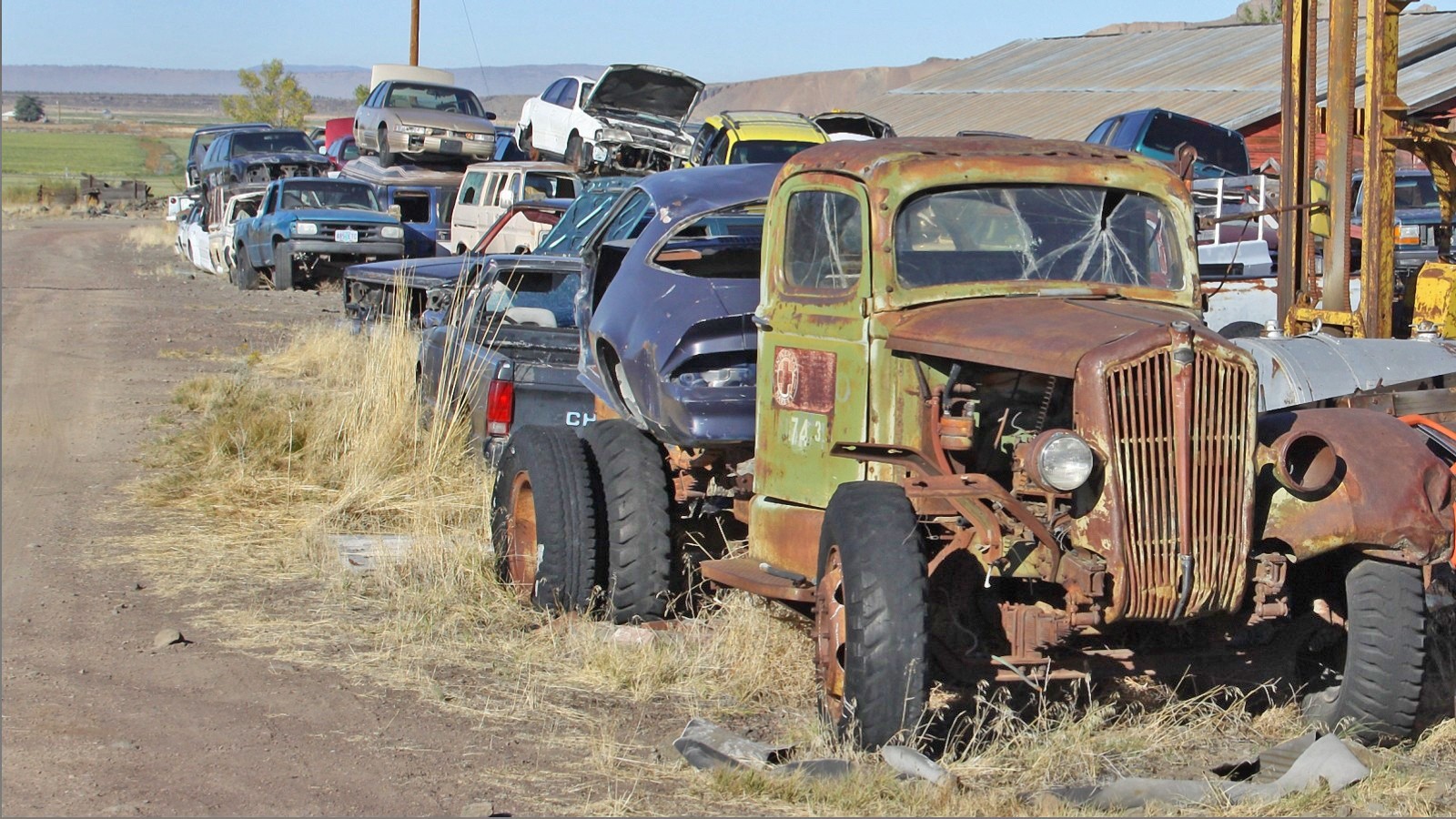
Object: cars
352 65 495 172
514 63 703 177
682 109 832 168
167 107 1456 753
